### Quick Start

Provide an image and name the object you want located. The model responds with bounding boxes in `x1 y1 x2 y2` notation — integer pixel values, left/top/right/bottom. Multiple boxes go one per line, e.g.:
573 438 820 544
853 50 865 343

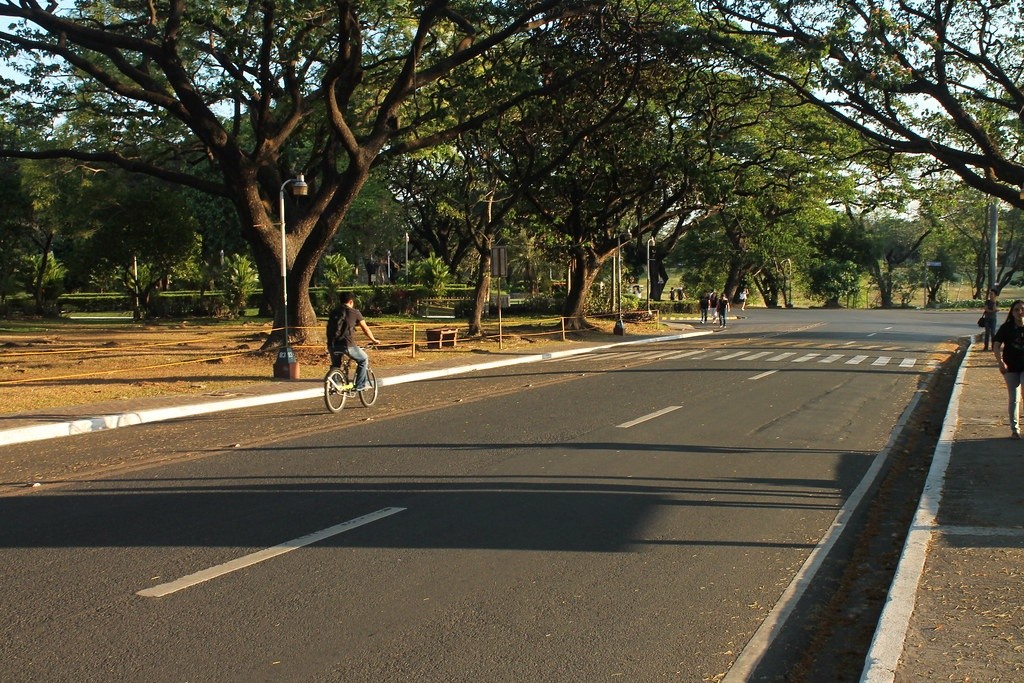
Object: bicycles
321 339 381 413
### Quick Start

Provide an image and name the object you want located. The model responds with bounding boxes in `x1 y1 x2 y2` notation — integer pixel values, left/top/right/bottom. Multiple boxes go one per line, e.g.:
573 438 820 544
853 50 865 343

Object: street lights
402 232 412 286
387 251 392 285
645 236 656 318
271 175 308 382
613 227 632 336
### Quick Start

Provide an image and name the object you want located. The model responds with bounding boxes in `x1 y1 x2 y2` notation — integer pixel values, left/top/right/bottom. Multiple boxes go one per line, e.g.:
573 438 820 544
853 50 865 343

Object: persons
699 290 710 324
709 292 718 324
325 291 382 392
739 284 749 311
983 289 999 352
708 289 714 300
992 299 1024 439
675 286 685 301
668 287 676 301
716 293 731 330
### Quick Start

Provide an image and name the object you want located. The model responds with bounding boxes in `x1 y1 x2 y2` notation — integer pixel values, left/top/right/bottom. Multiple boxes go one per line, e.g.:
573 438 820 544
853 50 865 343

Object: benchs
423 326 459 349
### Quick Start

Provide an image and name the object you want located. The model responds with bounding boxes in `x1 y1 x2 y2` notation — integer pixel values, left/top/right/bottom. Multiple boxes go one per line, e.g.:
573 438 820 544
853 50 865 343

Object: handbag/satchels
977 318 994 328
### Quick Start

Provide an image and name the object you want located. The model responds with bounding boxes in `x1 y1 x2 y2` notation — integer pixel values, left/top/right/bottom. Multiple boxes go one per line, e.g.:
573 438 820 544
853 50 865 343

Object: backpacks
326 306 357 344
